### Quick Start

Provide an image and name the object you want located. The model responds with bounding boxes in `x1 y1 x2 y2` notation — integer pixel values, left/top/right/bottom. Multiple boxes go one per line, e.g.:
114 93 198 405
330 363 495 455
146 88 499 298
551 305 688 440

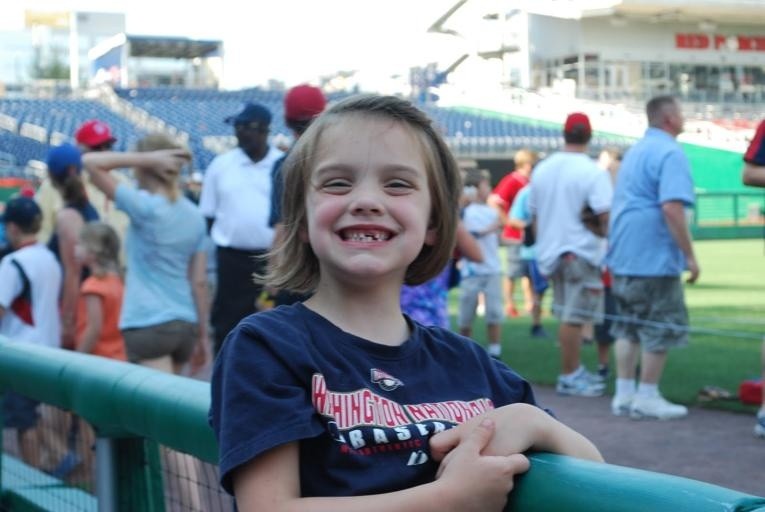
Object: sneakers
630 383 688 419
610 379 636 416
571 365 603 383
753 404 764 436
557 375 605 397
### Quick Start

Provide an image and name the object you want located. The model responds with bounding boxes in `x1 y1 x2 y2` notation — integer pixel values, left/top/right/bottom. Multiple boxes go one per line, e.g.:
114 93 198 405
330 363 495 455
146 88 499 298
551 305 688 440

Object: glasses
91 145 112 151
234 122 259 130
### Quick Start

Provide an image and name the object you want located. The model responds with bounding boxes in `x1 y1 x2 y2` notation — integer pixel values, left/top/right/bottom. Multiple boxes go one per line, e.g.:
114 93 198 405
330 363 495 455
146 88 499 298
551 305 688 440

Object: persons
269 85 326 304
197 101 288 351
77 223 130 362
216 97 608 512
744 115 765 440
529 114 615 397
47 143 99 279
401 213 482 334
485 146 538 317
458 170 506 362
81 129 210 373
0 196 84 478
609 96 698 421
596 146 623 375
33 124 133 265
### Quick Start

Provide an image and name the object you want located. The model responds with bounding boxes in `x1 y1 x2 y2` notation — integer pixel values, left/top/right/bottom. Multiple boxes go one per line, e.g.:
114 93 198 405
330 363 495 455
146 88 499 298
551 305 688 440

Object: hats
75 121 116 147
223 103 273 124
0 198 42 223
47 143 82 175
565 114 591 134
283 84 327 119
